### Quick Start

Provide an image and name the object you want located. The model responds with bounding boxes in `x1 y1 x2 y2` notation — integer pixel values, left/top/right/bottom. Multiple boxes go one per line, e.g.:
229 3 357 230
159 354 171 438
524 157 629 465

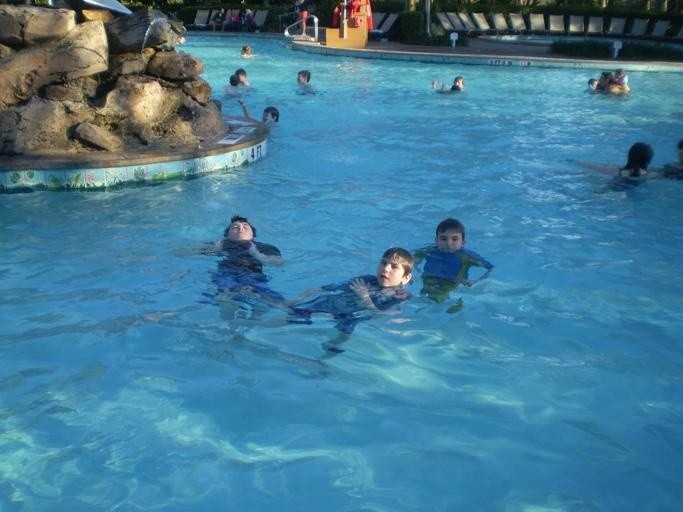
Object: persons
414 216 495 313
298 70 311 85
239 100 279 122
288 246 416 360
229 69 250 87
564 139 682 177
213 7 253 32
589 69 629 96
240 46 250 55
136 213 311 329
432 76 464 90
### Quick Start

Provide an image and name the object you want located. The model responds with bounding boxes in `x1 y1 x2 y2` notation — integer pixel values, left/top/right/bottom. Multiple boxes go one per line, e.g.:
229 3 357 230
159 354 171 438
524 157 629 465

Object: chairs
369 13 400 43
372 12 388 29
185 8 269 34
